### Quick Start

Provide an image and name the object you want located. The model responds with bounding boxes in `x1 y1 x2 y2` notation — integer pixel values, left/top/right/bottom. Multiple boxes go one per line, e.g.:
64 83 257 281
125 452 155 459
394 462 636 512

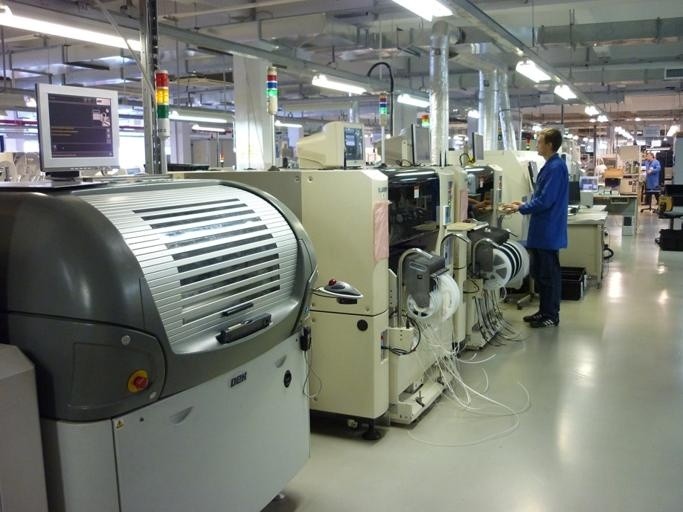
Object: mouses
461 218 479 223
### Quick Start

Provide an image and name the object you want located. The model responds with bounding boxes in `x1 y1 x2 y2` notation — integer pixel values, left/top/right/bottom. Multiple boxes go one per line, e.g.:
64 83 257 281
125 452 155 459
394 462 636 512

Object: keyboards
447 221 476 230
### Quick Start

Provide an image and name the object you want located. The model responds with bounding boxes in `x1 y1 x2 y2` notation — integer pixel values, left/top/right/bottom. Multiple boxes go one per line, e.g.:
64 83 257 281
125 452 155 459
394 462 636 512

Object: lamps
19 96 305 136
311 72 369 96
0 4 146 55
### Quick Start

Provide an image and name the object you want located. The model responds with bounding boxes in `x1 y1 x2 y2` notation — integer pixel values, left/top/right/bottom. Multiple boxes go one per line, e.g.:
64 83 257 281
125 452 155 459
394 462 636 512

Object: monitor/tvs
410 123 432 166
472 132 485 163
34 82 120 171
580 176 600 193
294 121 367 168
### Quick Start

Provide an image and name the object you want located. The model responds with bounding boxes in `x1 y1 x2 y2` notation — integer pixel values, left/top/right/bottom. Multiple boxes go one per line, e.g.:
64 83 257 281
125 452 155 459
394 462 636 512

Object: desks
558 169 642 284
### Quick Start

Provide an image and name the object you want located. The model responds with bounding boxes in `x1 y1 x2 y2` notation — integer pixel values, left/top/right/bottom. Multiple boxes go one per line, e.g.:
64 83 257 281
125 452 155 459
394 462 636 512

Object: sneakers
530 316 559 327
524 312 543 322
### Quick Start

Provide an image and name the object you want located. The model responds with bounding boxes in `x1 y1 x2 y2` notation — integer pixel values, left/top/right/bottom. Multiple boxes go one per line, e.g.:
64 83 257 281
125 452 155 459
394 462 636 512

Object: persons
640 152 662 206
502 128 569 330
